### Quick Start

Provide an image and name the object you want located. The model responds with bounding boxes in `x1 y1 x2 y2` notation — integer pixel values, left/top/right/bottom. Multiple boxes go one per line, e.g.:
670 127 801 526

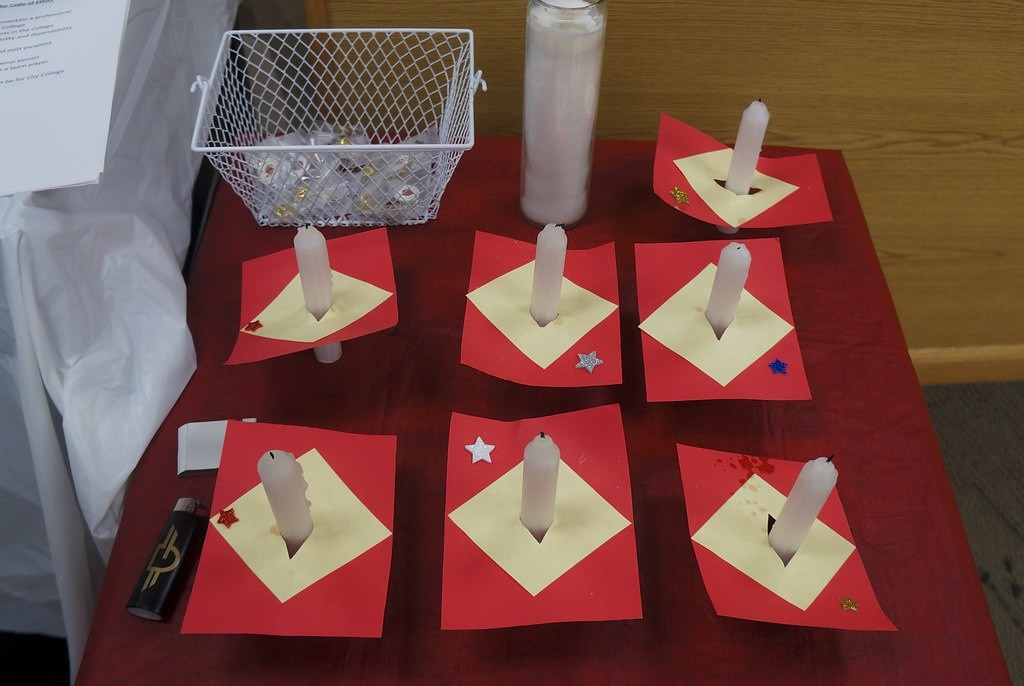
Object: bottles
519 0 608 228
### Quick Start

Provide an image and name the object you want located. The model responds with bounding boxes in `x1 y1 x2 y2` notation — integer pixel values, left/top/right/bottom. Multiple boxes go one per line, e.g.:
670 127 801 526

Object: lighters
125 498 210 622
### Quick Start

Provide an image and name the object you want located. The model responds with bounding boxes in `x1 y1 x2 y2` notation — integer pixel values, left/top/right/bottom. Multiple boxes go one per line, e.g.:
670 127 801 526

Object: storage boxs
186 30 490 221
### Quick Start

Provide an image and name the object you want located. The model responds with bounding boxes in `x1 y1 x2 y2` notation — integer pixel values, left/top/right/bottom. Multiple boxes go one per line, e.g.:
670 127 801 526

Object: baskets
189 28 488 226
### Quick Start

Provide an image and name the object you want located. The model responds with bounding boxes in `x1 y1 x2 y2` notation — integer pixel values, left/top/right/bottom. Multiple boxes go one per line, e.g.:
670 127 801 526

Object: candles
290 220 348 370
257 449 314 548
529 220 572 331
517 429 559 537
717 96 769 241
708 237 754 336
767 454 838 562
